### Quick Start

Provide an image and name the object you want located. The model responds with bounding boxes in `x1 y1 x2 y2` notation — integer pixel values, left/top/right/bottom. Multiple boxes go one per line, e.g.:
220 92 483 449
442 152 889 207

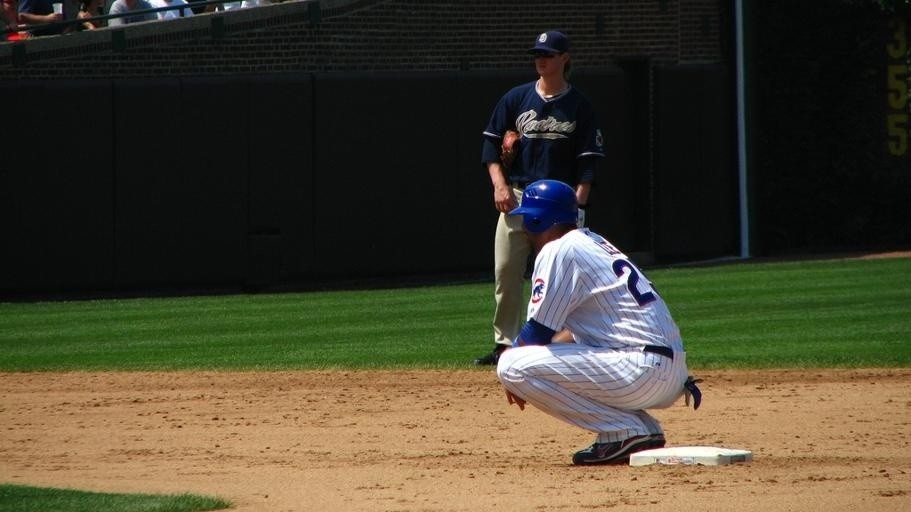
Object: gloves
682 374 705 411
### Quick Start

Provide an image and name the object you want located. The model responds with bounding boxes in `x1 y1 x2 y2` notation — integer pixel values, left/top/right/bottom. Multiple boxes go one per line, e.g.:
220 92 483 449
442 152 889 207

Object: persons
495 179 691 467
473 32 608 366
1 1 284 43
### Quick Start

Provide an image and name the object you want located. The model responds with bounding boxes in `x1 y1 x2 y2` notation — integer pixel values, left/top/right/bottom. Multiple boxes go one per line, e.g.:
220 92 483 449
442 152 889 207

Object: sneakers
571 432 667 466
473 343 514 367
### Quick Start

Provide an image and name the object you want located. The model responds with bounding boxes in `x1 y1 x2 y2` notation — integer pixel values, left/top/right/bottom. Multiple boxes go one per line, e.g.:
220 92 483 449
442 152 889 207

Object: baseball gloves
500 130 522 168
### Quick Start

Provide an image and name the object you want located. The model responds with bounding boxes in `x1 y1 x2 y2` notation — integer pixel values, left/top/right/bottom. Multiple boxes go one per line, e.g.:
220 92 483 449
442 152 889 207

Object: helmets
507 179 581 234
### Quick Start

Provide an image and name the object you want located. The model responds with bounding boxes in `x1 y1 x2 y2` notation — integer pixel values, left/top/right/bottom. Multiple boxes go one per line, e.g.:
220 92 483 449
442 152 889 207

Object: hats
527 30 570 60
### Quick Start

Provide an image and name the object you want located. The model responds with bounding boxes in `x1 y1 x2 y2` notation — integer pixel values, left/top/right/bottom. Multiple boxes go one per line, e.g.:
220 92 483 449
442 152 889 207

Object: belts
634 345 674 361
